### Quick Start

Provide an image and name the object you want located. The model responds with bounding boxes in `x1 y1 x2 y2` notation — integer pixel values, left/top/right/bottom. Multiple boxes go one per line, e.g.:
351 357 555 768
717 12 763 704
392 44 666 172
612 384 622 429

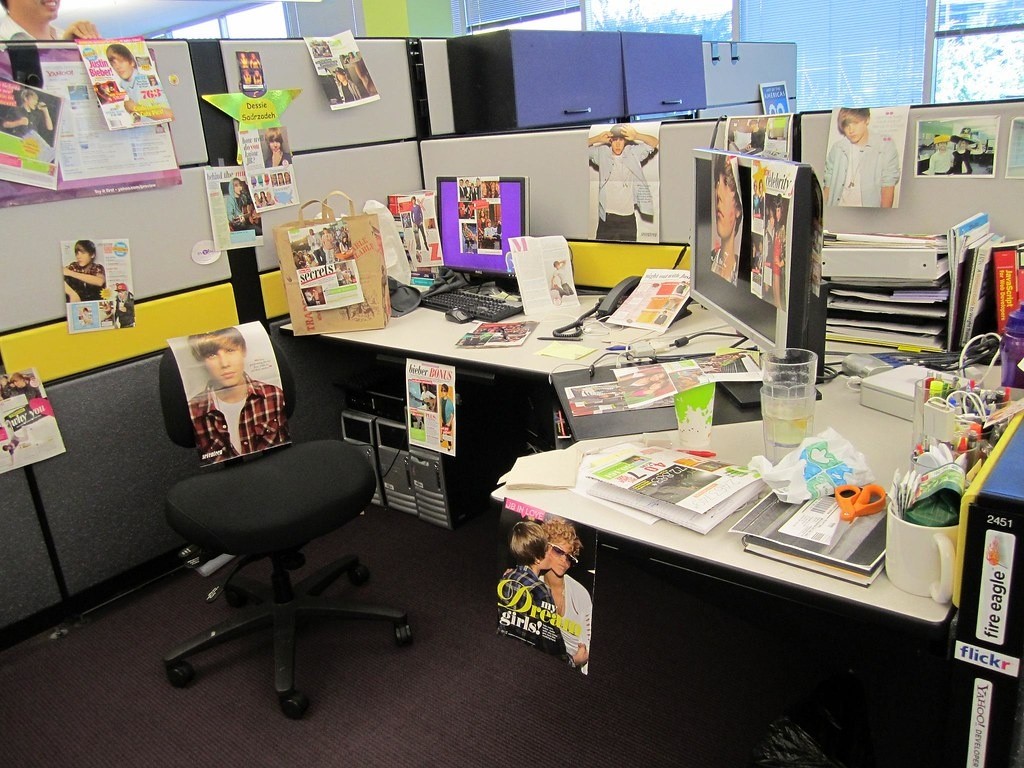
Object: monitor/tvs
436 177 526 293
688 149 828 411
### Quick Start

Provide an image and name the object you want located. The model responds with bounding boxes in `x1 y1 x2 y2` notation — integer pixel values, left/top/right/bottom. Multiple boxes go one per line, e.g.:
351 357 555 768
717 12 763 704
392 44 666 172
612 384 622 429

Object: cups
885 501 961 603
911 375 1016 478
759 347 818 467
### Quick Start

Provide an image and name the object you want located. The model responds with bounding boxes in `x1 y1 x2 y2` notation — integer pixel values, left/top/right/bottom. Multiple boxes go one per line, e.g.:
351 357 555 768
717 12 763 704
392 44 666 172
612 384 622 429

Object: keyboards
418 290 523 322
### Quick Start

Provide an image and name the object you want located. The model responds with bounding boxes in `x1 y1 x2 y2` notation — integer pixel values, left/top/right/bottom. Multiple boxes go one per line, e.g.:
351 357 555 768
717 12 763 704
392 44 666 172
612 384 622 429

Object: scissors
828 484 886 552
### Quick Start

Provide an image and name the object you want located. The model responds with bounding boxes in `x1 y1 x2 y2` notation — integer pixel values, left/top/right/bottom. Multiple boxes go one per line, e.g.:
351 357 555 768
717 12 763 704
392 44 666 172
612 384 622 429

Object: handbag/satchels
315 200 412 287
273 191 392 336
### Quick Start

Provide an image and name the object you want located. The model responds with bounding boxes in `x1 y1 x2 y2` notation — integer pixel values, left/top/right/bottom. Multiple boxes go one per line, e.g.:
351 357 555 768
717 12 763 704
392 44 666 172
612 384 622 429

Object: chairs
159 335 413 721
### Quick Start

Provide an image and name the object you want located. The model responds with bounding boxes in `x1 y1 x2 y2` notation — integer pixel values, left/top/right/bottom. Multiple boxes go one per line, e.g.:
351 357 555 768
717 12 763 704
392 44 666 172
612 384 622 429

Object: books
740 490 887 589
582 444 767 535
816 212 1024 349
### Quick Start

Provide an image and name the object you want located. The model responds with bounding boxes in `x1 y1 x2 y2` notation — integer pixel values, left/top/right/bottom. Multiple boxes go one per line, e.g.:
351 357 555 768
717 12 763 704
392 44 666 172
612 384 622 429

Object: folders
821 248 950 279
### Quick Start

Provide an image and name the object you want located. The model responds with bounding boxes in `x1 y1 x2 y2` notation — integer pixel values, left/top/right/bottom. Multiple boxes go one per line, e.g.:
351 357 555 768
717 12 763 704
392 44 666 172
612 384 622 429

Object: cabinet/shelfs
447 28 707 134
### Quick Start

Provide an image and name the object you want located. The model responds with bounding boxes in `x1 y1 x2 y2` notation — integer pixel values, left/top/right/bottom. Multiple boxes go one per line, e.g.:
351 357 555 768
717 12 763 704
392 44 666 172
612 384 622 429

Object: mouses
445 307 474 323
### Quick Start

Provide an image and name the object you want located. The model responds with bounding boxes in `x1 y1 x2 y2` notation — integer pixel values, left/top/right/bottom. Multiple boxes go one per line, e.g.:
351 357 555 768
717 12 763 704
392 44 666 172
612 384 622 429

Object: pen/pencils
500 327 510 341
537 337 584 341
927 370 933 378
676 449 716 457
970 379 975 389
943 382 948 398
936 372 942 379
1004 388 1010 403
949 377 959 395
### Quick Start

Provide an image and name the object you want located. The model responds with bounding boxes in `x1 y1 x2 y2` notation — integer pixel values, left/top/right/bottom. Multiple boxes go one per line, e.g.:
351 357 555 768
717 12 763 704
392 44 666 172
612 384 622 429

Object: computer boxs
342 407 475 530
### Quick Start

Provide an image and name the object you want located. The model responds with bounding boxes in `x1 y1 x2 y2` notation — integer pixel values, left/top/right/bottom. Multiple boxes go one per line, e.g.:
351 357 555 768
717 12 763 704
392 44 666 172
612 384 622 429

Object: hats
333 67 346 74
115 284 127 291
608 125 625 138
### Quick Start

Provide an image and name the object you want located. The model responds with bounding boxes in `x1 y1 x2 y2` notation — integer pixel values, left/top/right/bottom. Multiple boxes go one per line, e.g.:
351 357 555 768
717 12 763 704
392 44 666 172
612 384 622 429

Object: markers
930 381 942 399
925 378 935 402
967 430 978 473
957 436 967 456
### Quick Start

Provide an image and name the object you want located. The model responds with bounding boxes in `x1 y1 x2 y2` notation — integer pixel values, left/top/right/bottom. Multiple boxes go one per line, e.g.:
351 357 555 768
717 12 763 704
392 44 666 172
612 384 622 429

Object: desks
278 294 1002 768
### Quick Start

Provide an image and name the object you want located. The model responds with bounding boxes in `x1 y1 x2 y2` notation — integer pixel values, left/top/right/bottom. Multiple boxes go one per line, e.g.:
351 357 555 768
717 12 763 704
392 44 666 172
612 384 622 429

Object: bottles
1000 302 1024 388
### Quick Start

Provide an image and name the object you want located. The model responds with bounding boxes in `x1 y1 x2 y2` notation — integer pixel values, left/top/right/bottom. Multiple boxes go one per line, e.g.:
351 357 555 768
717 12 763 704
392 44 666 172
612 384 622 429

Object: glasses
546 541 578 567
440 390 446 392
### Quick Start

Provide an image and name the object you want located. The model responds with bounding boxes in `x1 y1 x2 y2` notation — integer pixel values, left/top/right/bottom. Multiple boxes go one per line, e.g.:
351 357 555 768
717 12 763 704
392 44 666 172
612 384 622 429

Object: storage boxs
860 365 969 423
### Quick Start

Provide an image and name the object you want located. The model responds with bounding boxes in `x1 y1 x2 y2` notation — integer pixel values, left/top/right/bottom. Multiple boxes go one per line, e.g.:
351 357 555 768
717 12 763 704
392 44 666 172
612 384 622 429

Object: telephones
596 275 694 328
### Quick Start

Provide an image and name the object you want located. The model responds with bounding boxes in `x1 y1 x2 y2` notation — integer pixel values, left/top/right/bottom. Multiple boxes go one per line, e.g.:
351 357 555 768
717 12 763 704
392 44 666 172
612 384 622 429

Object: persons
711 109 973 308
223 126 354 306
106 44 175 126
408 384 454 454
461 257 745 419
0 372 46 465
0 0 101 40
2 89 53 139
188 327 289 466
239 39 377 103
62 240 134 330
410 176 501 252
497 519 592 676
588 123 660 239
